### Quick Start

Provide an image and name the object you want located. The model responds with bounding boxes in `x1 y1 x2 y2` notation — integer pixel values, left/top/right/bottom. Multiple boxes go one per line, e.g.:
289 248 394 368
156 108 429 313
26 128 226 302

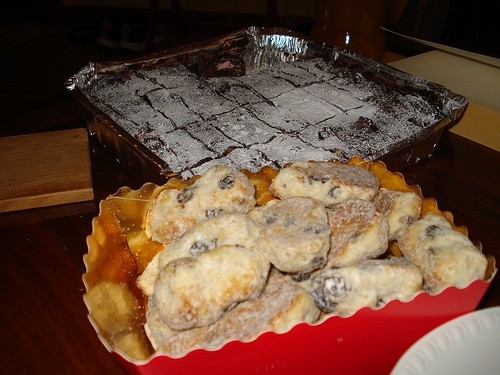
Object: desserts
90 51 448 181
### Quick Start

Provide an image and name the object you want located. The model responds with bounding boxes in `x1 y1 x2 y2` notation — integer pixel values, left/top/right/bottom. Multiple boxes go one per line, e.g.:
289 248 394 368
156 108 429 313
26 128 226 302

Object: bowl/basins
84 151 500 375
387 306 499 375
